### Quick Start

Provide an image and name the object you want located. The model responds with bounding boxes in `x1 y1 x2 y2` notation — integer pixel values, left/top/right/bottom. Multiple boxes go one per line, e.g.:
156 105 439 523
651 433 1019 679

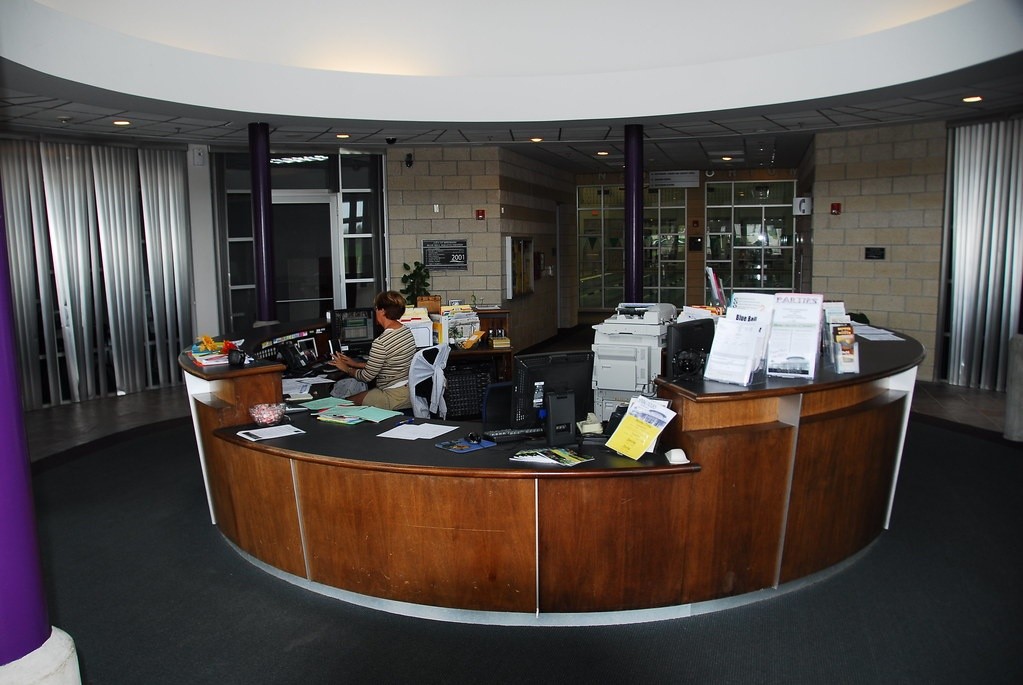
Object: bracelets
347 368 352 375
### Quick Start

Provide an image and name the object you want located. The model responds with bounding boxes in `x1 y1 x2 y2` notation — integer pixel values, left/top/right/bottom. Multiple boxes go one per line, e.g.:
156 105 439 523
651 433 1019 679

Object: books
298 396 404 425
509 446 595 467
185 336 254 368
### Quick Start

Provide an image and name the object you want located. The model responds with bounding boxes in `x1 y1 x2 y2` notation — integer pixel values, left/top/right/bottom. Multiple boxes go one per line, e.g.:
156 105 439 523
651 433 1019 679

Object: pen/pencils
394 417 415 426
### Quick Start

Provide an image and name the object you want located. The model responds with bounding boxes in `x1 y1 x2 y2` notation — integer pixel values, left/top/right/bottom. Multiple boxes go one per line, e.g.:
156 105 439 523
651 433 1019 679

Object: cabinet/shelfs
441 306 513 389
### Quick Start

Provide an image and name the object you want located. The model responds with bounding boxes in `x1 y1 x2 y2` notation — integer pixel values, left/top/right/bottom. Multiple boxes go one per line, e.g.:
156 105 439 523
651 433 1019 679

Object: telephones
279 344 311 371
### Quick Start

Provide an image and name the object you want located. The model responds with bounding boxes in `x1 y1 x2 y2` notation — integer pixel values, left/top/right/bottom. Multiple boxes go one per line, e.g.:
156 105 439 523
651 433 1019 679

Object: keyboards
482 424 567 443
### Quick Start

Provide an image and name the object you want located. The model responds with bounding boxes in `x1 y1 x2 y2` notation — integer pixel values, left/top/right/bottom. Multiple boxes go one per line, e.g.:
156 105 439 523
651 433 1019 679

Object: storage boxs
417 295 441 314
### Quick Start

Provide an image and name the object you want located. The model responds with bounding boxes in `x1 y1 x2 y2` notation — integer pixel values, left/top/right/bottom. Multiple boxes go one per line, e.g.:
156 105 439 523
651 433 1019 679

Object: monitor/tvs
332 308 376 348
509 351 596 448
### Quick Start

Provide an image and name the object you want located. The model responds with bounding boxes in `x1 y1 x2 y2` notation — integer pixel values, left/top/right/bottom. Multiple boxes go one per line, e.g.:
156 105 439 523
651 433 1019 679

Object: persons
327 290 417 411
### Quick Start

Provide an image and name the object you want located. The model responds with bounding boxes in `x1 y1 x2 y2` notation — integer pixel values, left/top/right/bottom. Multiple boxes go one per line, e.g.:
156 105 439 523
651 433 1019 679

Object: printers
591 303 676 422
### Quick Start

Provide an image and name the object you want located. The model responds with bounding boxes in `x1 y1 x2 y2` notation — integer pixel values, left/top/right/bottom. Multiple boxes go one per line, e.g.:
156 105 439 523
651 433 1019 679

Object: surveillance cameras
386 137 397 145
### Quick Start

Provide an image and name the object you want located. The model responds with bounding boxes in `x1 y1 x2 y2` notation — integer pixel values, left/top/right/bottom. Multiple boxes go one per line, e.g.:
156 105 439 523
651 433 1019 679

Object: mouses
465 432 481 443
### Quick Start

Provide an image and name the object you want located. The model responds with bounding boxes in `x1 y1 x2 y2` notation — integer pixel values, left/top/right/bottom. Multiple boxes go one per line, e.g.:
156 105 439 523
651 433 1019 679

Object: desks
213 379 673 469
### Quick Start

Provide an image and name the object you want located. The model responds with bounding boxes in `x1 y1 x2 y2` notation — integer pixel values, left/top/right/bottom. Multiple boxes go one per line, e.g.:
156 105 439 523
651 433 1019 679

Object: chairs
392 345 452 421
